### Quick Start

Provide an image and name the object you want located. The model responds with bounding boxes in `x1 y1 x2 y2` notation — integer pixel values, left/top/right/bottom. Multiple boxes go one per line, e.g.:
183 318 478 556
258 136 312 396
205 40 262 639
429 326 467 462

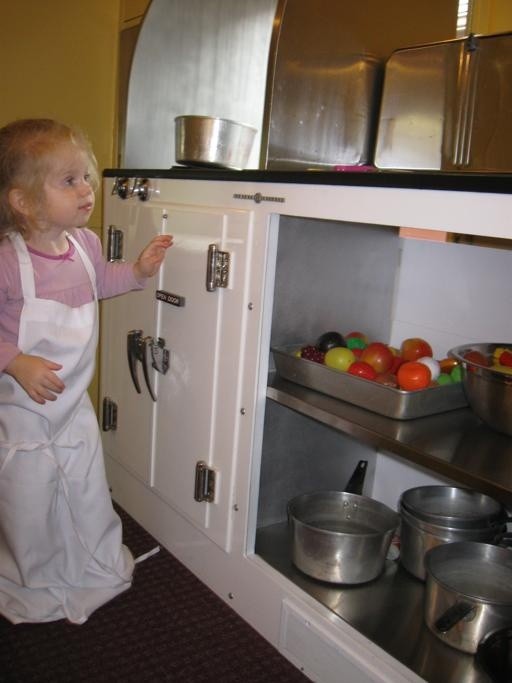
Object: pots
392 485 511 582
420 542 511 653
288 459 397 583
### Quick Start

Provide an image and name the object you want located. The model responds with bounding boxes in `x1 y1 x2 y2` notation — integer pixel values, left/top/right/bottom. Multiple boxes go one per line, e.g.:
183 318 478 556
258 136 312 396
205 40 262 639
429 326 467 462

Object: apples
460 348 489 371
345 331 369 356
451 364 465 383
360 342 394 374
375 374 398 390
435 370 451 385
400 338 433 363
348 360 375 382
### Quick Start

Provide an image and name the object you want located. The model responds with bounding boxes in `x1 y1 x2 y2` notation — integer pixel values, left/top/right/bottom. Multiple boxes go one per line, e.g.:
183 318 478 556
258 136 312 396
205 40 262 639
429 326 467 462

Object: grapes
300 345 325 364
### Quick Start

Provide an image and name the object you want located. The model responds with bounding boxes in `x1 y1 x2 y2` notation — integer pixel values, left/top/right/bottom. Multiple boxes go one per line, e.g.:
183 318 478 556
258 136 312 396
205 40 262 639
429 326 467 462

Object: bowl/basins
173 113 256 171
444 341 512 440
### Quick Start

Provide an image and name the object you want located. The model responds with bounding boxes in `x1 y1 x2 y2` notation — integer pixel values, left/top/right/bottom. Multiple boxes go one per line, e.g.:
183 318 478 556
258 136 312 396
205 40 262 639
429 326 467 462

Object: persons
1 118 174 626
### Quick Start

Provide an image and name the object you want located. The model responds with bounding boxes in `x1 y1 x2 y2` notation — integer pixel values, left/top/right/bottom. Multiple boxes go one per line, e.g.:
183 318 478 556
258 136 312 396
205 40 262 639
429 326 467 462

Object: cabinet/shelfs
94 173 401 552
240 169 512 683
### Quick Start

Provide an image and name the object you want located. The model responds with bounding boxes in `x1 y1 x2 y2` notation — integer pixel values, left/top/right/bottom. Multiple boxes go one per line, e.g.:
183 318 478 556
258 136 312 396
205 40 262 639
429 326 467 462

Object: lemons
325 347 357 372
